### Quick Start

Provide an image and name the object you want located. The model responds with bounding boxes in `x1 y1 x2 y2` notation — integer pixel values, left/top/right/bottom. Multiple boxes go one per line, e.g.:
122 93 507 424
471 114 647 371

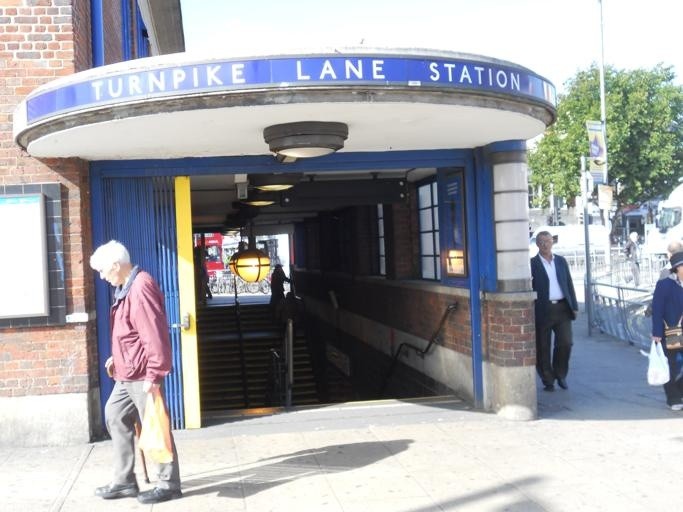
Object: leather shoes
555 377 568 389
544 385 555 391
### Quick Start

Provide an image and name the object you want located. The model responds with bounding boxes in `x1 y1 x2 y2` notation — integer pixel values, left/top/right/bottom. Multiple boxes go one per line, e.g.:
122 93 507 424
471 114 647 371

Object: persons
269 263 291 308
650 252 683 411
623 231 643 288
644 241 683 319
528 231 579 392
89 239 183 503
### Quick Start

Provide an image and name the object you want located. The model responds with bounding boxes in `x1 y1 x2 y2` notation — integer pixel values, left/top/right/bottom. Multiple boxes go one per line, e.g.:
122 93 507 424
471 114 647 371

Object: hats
668 252 683 272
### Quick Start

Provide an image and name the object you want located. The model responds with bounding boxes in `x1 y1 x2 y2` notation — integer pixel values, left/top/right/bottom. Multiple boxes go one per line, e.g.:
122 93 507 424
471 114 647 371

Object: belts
546 300 562 303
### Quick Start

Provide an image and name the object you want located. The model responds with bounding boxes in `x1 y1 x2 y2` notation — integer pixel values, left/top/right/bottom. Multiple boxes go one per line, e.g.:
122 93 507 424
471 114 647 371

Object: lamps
265 123 349 159
226 224 272 281
241 174 304 206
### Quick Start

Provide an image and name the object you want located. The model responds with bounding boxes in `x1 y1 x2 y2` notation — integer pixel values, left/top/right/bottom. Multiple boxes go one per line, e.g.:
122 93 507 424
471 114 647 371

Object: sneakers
137 488 181 503
667 403 683 411
94 483 139 498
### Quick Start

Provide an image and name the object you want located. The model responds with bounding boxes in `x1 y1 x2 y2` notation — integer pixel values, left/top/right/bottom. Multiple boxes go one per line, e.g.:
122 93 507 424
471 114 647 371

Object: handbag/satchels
664 327 683 350
